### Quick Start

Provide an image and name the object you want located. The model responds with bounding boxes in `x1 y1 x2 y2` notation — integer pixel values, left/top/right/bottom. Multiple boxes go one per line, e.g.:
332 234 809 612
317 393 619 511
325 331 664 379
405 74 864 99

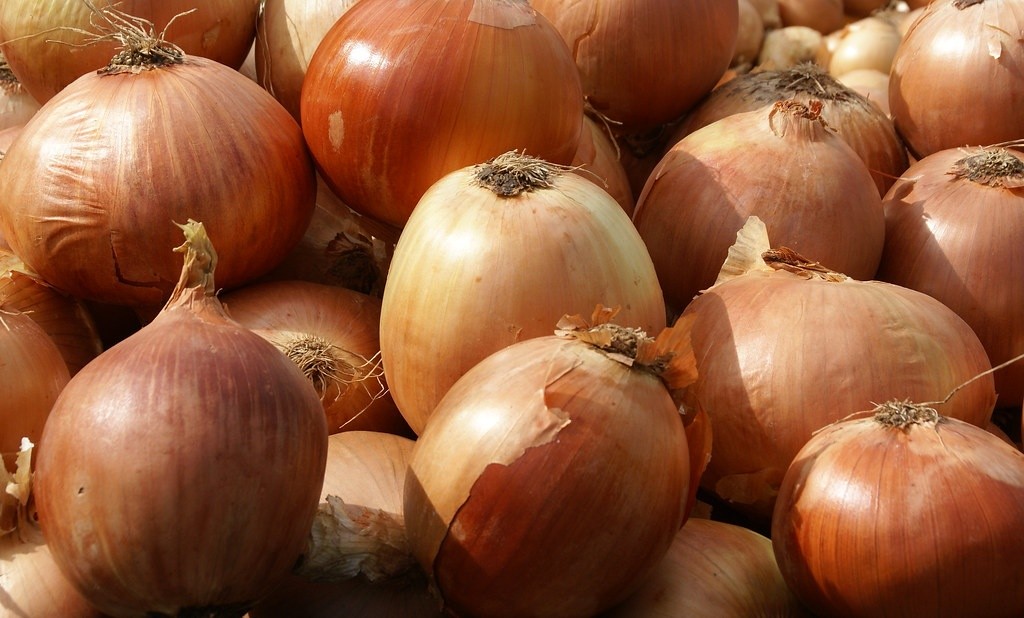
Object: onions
0 0 1024 618
